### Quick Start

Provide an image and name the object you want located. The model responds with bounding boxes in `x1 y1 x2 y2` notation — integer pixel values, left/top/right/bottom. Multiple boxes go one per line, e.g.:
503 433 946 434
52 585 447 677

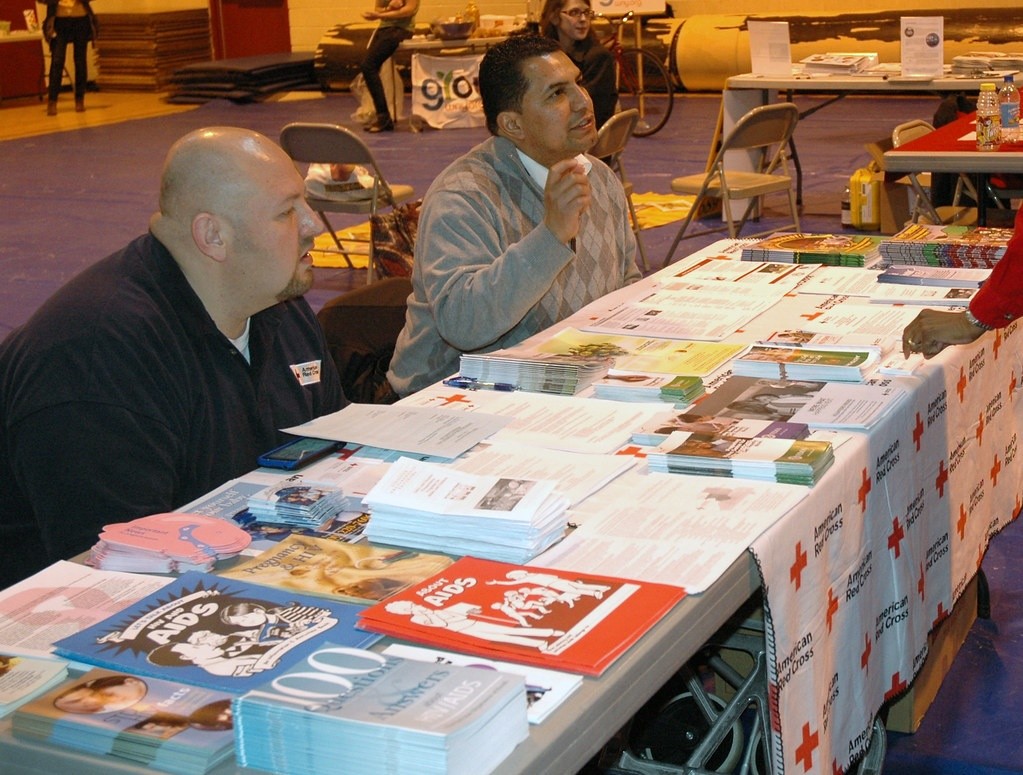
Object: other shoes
73 97 85 113
48 100 58 114
363 119 394 132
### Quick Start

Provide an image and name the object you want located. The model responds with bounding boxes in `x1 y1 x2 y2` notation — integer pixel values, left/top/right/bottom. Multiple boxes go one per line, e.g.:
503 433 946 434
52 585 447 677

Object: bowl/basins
431 17 475 39
427 33 435 39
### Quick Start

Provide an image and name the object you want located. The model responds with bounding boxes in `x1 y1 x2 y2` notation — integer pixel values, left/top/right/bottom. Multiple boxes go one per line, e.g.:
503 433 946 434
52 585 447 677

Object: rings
907 338 915 345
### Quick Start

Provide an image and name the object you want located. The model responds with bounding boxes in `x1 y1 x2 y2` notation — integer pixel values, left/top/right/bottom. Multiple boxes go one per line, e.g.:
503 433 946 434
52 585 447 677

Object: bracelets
965 309 993 332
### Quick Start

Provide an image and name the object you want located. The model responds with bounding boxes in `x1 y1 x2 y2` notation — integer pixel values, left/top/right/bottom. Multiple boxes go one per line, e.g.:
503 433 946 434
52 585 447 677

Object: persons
40 0 98 118
903 197 1023 361
364 0 419 132
378 38 645 396
537 0 619 168
0 127 354 607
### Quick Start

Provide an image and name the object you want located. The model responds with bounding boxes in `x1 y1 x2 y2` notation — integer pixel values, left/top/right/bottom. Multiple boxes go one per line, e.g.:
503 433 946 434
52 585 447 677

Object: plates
956 70 1020 77
888 74 935 81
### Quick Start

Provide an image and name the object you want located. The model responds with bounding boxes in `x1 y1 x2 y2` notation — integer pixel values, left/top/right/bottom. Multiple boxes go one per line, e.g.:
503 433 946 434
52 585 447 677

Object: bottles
456 12 463 23
841 184 855 228
465 3 481 28
977 83 1002 152
998 74 1022 143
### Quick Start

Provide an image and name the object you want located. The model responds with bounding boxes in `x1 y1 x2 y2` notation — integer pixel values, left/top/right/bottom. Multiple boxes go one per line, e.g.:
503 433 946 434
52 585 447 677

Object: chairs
891 120 1017 229
581 107 650 272
660 101 803 268
277 122 413 290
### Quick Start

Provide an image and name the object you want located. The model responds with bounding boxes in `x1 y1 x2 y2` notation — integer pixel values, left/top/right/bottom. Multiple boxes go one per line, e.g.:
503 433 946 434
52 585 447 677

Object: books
0 222 1023 774
799 50 1023 77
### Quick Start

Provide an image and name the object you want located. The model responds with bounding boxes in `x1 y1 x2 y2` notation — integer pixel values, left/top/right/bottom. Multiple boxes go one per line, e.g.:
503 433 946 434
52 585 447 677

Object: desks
387 34 526 133
883 82 1023 229
717 62 1023 223
0 230 1023 775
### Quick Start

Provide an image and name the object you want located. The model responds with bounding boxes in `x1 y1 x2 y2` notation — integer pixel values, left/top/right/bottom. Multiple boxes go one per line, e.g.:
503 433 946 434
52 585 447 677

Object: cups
0 21 12 36
24 9 40 33
495 19 504 28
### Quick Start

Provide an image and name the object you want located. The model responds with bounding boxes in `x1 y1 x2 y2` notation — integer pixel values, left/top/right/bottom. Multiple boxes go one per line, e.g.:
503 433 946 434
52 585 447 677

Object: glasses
557 7 595 20
